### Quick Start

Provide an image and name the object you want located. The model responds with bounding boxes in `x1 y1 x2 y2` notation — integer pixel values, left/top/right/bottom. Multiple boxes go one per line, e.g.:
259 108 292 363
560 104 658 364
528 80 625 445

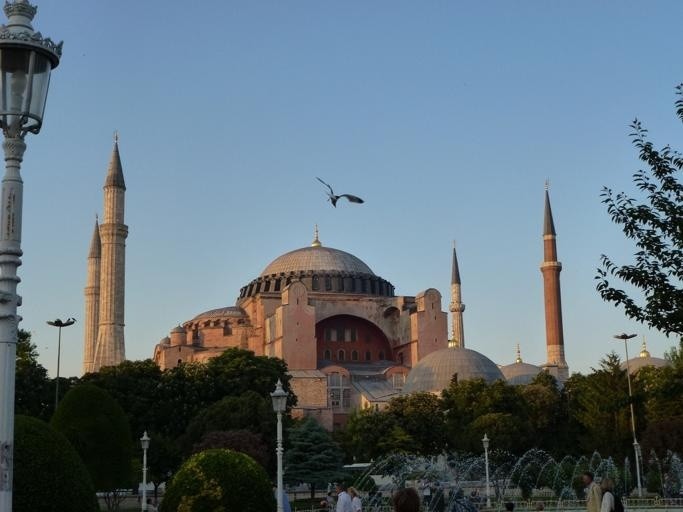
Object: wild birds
316 177 364 208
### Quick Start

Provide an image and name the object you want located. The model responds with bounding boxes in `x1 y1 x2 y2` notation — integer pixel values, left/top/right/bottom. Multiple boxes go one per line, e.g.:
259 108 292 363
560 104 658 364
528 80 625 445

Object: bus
343 461 397 490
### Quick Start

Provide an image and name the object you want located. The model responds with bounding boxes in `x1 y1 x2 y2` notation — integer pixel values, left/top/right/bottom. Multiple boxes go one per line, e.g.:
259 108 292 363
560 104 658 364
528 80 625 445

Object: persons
392 488 421 512
346 487 363 512
146 498 157 512
335 483 352 511
326 492 334 511
533 500 546 511
475 487 481 503
272 485 290 512
582 471 601 512
600 477 615 511
470 488 476 502
505 502 514 511
418 478 431 507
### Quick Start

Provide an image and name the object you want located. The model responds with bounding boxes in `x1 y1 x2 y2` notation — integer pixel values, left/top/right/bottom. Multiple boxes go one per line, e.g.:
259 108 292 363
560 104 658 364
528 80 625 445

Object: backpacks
601 490 624 512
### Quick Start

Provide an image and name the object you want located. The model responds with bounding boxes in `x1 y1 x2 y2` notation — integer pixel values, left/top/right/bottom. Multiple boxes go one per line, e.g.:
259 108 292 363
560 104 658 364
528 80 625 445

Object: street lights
614 333 643 496
479 431 492 508
269 377 289 511
46 317 76 412
138 429 151 511
